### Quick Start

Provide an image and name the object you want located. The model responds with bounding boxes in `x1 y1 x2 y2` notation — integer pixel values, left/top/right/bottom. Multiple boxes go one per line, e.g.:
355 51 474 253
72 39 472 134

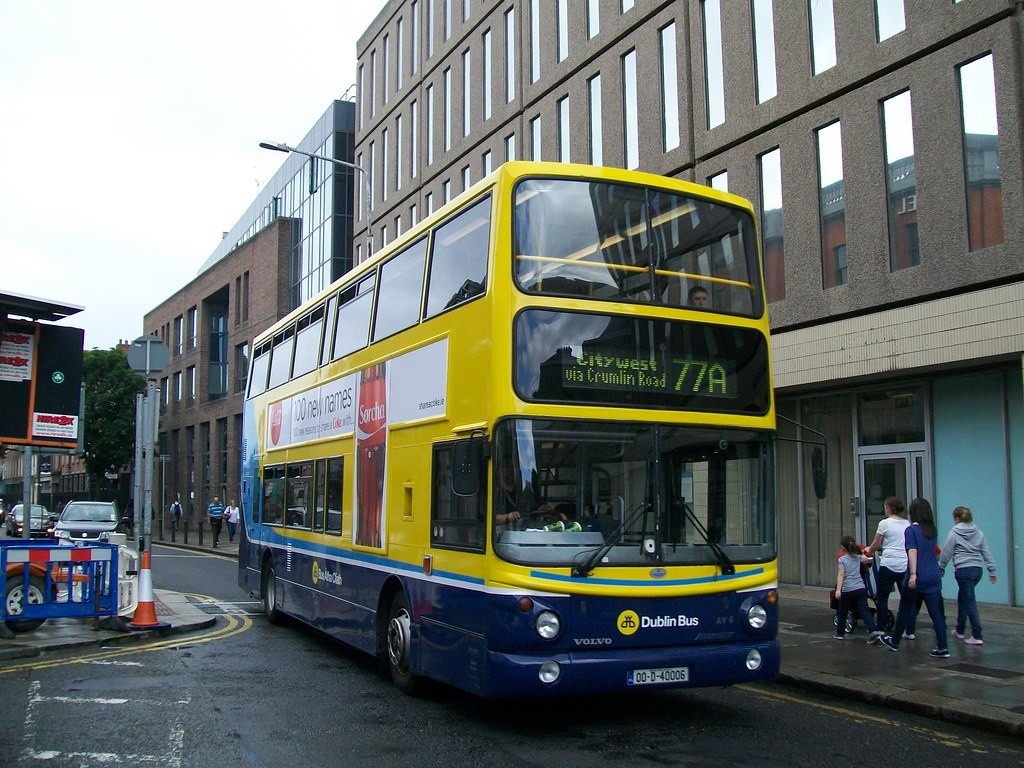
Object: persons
477 460 565 532
56 501 63 515
0 498 22 522
125 498 155 536
689 287 709 308
863 498 917 644
938 506 998 645
206 496 224 545
831 535 877 640
880 497 949 658
170 498 183 532
224 499 240 543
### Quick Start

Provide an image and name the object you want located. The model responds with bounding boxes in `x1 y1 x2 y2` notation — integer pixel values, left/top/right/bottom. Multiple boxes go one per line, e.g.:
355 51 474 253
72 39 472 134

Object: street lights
258 141 373 260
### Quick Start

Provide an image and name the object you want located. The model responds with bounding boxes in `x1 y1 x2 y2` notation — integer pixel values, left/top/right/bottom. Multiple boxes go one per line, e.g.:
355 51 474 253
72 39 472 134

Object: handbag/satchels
223 512 231 520
121 513 130 523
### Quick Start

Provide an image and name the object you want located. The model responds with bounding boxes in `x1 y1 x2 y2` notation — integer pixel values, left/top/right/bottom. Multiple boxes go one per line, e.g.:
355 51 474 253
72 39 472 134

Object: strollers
828 544 895 632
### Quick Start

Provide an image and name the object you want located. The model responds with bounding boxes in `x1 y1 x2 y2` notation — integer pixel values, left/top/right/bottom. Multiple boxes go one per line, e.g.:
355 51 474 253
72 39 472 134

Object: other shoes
865 628 886 636
867 633 883 645
230 541 234 544
832 633 845 640
901 630 915 640
216 541 219 545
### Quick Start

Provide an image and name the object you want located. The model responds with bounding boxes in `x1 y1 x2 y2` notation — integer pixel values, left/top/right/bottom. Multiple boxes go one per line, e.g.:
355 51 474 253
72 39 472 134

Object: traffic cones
123 549 171 630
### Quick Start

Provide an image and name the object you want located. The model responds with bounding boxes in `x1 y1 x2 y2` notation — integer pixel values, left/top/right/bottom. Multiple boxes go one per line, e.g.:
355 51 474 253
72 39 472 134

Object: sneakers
929 647 951 659
964 636 984 645
879 634 899 652
951 629 965 639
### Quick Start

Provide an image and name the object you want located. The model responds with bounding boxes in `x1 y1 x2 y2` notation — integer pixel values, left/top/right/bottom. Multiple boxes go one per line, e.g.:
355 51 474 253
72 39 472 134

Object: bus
237 160 831 709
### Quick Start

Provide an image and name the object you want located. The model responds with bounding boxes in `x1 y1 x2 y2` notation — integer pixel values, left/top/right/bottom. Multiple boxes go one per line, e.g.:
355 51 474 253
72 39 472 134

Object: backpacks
173 502 182 515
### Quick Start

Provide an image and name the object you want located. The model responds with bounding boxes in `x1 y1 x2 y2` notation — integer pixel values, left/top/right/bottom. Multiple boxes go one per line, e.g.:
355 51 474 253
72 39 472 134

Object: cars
6 504 58 538
0 498 7 527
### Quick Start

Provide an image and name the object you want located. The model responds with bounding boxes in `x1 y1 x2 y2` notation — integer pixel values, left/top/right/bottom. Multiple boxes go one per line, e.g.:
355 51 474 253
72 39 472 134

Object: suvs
48 498 131 564
